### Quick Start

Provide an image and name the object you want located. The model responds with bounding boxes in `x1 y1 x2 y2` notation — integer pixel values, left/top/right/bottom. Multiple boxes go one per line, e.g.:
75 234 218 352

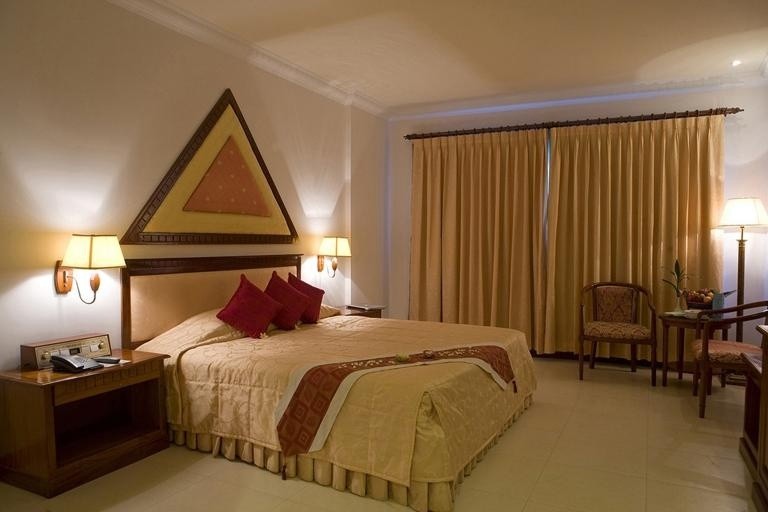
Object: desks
751 324 768 511
658 315 731 394
740 352 763 480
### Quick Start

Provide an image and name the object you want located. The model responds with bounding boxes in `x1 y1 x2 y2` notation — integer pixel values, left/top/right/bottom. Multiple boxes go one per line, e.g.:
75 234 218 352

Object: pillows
216 274 284 338
135 303 340 427
262 271 309 330
288 272 326 323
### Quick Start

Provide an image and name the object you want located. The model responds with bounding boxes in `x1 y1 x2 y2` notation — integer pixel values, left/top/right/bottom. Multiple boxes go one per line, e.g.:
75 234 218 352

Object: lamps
55 233 127 305
316 235 351 278
710 197 768 386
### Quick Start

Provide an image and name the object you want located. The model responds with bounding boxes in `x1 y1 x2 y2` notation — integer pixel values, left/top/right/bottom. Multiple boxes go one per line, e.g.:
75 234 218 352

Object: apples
688 288 713 303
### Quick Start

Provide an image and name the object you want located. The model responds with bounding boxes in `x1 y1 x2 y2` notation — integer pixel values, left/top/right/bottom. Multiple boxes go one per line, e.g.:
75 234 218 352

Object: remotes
94 357 120 364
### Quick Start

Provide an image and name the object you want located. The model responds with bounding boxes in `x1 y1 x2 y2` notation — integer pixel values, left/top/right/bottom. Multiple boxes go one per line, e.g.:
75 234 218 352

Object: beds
122 253 539 512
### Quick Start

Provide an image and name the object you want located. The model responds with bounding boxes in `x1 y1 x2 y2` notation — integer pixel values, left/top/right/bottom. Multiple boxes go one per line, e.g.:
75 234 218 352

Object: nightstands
334 305 382 319
0 347 172 499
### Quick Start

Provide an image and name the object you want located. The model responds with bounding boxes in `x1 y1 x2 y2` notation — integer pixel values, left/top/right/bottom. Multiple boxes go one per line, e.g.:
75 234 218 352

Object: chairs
690 300 768 418
578 281 656 386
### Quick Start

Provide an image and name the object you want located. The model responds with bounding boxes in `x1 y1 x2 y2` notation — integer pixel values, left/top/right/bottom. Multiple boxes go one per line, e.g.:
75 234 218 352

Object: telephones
49 354 104 372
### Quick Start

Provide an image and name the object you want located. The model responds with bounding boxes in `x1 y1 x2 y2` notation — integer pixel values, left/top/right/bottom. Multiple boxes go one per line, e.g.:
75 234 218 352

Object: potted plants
660 260 689 312
